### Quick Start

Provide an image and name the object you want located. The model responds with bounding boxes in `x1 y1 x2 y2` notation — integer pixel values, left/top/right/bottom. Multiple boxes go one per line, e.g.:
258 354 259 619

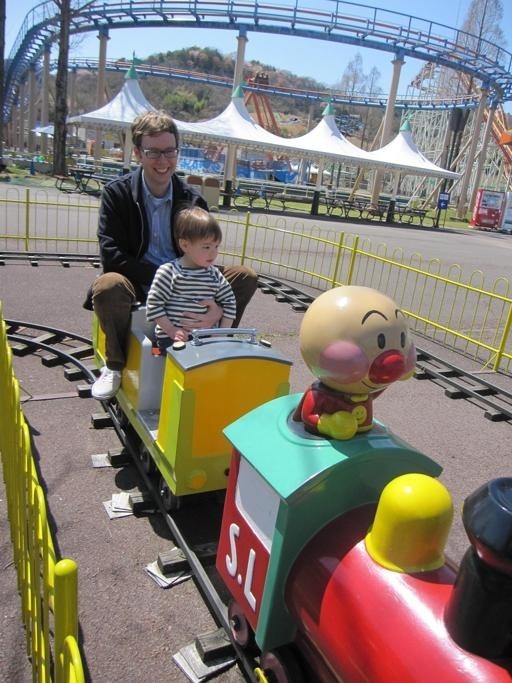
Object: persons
146 204 239 359
91 110 259 404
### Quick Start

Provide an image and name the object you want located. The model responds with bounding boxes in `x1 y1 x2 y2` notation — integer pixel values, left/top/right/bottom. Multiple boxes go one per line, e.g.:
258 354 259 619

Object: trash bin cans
202 175 220 211
184 174 203 196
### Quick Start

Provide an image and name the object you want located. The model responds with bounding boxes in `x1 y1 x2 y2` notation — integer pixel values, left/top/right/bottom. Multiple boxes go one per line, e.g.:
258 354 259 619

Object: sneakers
90 365 122 402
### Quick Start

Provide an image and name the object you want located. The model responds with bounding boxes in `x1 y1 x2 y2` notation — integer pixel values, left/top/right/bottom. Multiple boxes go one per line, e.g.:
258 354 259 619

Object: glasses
138 146 180 161
